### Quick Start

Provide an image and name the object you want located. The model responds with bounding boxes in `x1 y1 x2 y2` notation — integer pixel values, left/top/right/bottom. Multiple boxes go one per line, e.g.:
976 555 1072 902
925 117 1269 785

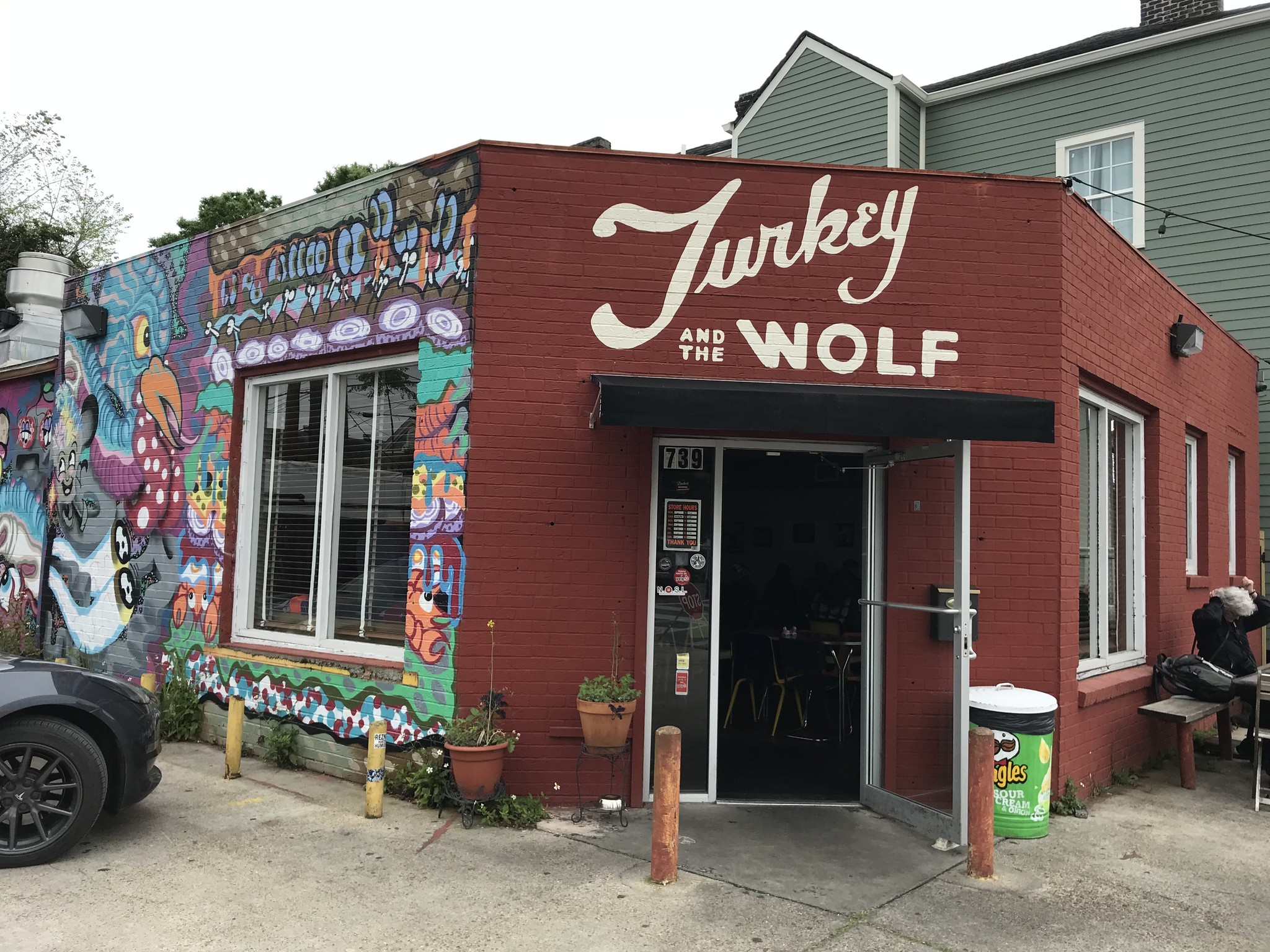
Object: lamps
58 297 109 342
1164 313 1206 361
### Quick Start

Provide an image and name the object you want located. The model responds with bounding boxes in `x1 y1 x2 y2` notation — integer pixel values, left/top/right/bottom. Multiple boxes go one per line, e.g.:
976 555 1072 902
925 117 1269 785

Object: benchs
1134 692 1243 793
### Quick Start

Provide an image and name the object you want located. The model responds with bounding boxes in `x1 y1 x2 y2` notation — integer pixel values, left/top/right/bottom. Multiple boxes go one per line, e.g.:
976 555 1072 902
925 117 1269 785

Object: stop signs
678 583 703 620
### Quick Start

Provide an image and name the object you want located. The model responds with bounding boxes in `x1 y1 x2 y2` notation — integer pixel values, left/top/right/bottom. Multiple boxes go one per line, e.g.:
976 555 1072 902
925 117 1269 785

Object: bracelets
1249 589 1256 595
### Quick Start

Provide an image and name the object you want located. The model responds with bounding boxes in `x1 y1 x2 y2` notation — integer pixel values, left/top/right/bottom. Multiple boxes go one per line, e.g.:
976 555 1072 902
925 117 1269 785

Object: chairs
820 630 861 711
1250 667 1270 818
719 630 812 738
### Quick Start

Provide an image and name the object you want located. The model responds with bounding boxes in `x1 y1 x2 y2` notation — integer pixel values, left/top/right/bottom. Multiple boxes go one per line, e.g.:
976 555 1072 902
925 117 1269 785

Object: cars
0 654 163 869
265 556 409 623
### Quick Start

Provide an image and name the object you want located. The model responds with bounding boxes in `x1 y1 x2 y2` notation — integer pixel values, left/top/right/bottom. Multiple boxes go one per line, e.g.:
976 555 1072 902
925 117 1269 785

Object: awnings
588 375 1055 443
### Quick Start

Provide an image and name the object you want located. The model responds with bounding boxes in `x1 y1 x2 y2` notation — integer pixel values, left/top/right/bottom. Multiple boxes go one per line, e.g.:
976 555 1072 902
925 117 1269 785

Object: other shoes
1236 738 1254 760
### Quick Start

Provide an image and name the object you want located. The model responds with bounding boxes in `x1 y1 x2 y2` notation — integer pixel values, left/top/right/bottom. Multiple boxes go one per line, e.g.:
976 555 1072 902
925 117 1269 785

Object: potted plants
574 608 644 759
438 617 522 802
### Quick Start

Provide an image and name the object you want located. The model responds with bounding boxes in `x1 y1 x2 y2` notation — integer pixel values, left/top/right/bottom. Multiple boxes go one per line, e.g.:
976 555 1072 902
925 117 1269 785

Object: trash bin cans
968 683 1059 841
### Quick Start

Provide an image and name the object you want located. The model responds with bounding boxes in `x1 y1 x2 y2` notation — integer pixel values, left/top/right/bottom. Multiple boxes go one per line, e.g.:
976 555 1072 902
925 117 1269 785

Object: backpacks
1152 653 1236 704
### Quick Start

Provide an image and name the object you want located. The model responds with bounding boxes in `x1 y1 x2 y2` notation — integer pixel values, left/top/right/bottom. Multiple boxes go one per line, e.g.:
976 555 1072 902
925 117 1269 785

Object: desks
728 627 863 742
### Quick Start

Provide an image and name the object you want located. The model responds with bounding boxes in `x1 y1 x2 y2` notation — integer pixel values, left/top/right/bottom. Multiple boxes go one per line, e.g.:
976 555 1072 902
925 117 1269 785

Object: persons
1192 575 1270 773
